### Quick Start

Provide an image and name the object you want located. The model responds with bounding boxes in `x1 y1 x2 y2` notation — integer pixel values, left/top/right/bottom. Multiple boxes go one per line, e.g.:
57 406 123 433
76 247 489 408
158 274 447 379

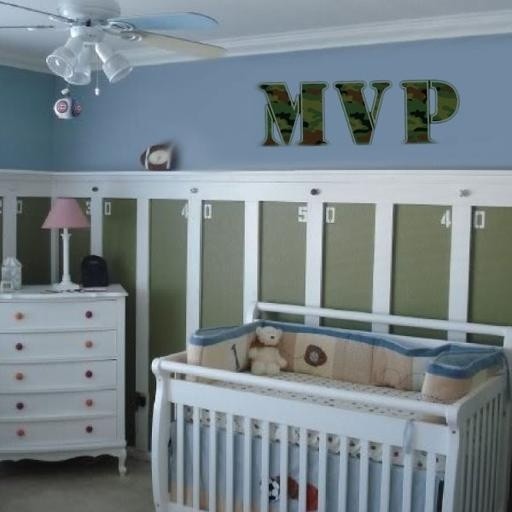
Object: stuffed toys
248 325 287 377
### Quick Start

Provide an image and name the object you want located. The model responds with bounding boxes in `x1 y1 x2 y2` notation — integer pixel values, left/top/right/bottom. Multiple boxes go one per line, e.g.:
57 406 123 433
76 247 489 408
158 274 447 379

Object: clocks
144 145 174 171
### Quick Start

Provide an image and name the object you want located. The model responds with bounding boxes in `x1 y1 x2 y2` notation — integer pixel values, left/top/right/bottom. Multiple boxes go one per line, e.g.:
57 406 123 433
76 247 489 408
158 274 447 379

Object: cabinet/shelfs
0 282 128 477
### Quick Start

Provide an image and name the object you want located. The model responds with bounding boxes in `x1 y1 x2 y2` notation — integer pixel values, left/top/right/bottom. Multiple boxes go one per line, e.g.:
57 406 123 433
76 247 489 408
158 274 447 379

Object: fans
0 0 228 58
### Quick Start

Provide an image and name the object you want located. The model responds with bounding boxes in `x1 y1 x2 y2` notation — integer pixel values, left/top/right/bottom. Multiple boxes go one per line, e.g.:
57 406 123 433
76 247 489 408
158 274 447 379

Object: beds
150 301 512 512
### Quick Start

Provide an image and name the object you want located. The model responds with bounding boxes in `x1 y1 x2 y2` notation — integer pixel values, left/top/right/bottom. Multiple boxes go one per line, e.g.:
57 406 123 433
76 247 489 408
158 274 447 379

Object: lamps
41 197 91 291
46 25 133 120
